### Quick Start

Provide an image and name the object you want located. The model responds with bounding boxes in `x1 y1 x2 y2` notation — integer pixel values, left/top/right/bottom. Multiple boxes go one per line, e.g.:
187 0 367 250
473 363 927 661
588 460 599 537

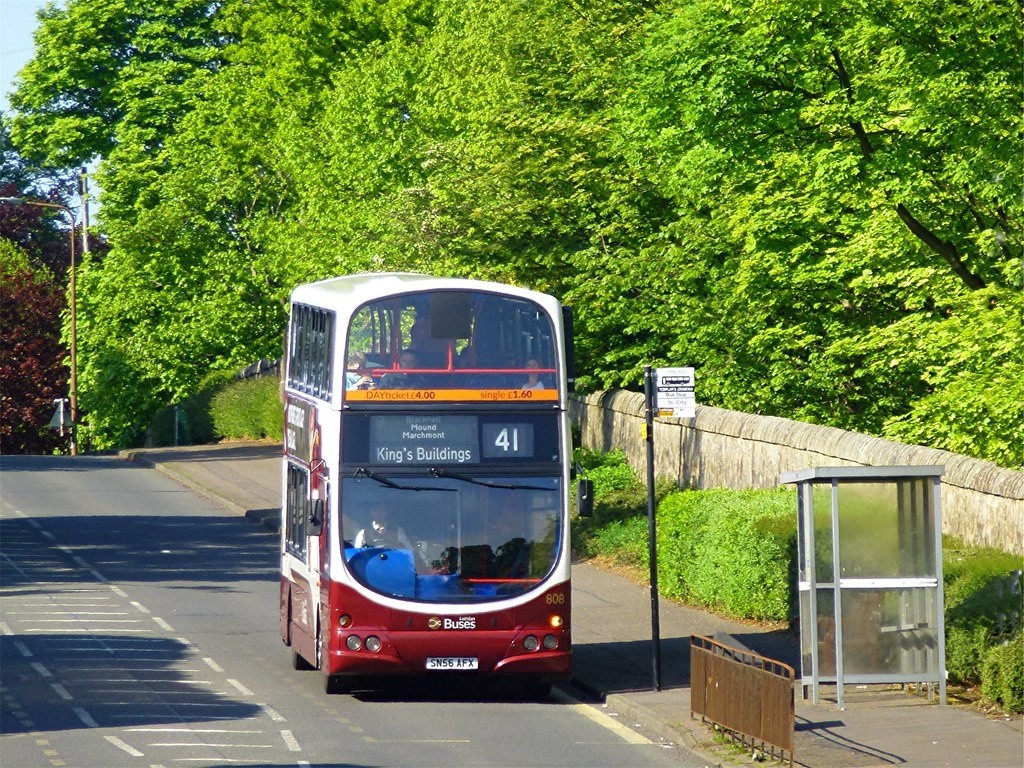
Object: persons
461 346 477 367
345 350 377 389
502 349 518 367
354 500 414 548
521 357 554 389
378 349 428 388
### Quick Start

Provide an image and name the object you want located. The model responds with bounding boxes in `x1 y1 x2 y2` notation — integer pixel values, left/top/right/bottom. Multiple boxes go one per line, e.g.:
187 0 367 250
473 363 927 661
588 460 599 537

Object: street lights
0 196 77 460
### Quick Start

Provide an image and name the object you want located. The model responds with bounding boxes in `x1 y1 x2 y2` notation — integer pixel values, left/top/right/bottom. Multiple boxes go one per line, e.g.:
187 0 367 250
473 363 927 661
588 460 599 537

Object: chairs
359 352 555 393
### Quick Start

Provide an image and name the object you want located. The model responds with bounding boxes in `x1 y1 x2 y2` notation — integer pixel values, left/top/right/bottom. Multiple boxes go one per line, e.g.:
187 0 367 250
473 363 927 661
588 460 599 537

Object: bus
279 269 595 700
